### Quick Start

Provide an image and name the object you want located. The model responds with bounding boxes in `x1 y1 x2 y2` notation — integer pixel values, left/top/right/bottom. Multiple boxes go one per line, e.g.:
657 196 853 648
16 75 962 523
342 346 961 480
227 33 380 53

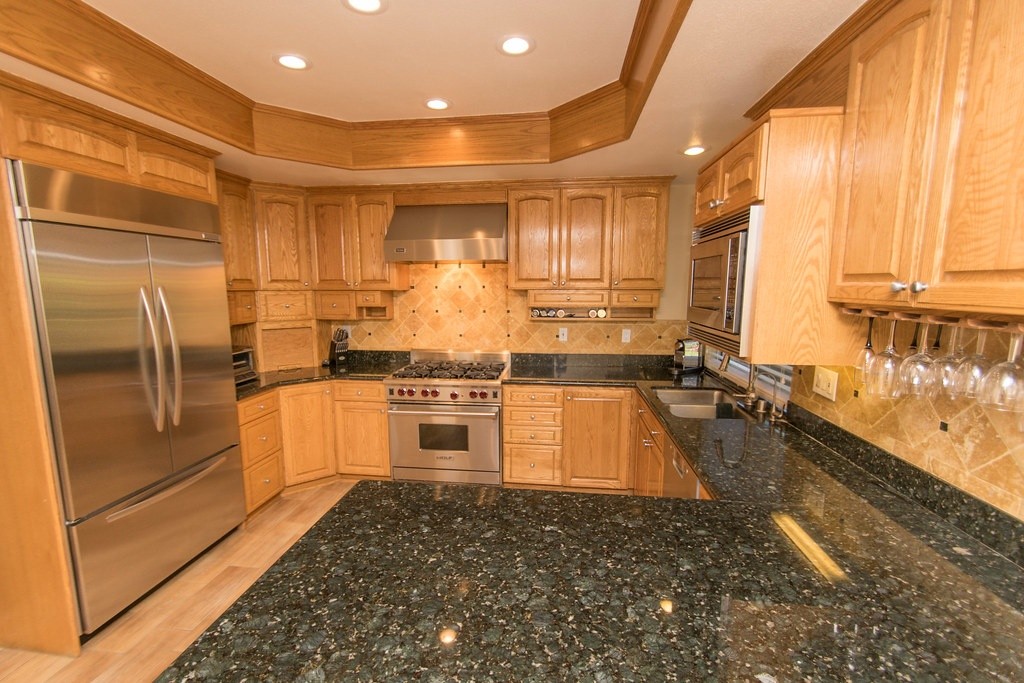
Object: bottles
532 308 606 317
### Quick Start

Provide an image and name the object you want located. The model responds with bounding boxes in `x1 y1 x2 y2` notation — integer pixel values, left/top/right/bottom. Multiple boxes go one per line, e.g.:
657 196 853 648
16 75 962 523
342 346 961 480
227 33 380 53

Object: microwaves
685 205 763 358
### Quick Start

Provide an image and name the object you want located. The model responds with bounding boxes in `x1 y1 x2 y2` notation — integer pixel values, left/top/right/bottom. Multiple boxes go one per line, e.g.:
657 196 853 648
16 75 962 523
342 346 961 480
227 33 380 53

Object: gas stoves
382 350 512 385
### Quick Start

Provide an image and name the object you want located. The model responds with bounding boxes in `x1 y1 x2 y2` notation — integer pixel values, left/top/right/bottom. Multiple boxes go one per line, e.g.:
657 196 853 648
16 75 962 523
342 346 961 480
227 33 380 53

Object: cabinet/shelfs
563 387 631 495
332 380 390 481
613 175 677 322
0 86 218 207
308 184 410 322
238 389 288 518
253 180 317 368
278 382 339 487
216 170 257 325
501 384 563 491
830 0 1024 322
507 179 612 322
692 107 844 227
633 390 666 497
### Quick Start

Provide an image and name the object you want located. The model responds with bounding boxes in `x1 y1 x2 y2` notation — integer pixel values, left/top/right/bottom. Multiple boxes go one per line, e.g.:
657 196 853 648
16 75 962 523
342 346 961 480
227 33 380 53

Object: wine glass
902 323 921 361
927 323 945 361
899 324 937 394
951 330 994 398
976 334 1024 412
853 318 877 368
923 326 960 395
954 327 970 359
864 320 906 399
992 330 1024 372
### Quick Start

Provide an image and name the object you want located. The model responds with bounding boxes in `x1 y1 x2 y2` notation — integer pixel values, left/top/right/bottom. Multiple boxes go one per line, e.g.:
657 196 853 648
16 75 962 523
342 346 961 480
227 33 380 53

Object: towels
715 403 733 419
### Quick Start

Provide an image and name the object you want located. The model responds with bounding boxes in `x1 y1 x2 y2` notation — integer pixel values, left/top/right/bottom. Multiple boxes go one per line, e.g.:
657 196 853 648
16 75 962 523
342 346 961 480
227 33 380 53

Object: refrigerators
1 155 248 645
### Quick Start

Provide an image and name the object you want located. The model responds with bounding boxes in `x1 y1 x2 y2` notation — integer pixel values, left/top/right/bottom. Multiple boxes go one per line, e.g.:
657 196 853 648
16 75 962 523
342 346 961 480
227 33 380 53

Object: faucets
750 371 786 422
719 353 757 406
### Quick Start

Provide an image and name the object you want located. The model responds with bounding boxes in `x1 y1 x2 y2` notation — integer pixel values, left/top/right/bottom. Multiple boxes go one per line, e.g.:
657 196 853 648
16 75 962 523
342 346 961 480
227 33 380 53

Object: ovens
385 384 503 486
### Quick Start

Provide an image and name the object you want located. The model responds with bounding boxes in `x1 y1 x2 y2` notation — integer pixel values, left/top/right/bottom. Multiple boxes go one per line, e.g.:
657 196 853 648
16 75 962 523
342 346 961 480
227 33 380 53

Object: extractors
382 203 507 262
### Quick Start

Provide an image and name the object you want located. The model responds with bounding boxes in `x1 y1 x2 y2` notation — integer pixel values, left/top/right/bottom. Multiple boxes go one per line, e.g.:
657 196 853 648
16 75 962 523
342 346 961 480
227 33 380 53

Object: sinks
668 404 752 419
653 388 739 404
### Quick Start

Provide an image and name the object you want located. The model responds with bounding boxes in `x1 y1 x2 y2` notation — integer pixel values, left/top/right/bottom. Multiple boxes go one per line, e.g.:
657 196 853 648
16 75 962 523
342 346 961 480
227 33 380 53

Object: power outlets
812 366 838 401
621 329 630 342
343 325 352 337
559 328 567 341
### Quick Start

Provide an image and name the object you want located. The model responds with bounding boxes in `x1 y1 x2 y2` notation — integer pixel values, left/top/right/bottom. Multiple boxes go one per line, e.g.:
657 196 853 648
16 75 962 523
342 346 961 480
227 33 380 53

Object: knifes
333 327 349 352
